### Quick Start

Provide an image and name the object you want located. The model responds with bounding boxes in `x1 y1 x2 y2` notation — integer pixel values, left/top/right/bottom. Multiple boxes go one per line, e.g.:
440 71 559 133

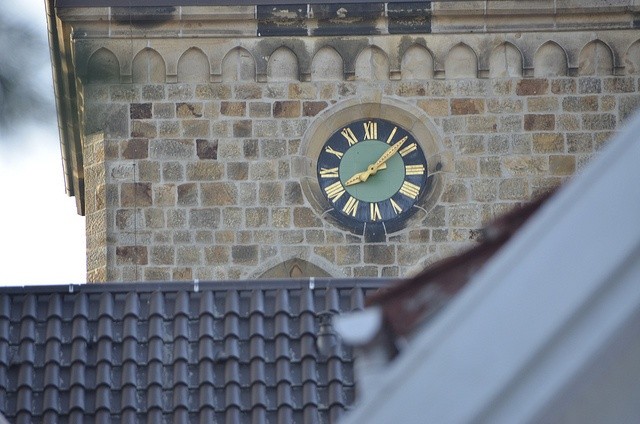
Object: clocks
317 116 429 223
